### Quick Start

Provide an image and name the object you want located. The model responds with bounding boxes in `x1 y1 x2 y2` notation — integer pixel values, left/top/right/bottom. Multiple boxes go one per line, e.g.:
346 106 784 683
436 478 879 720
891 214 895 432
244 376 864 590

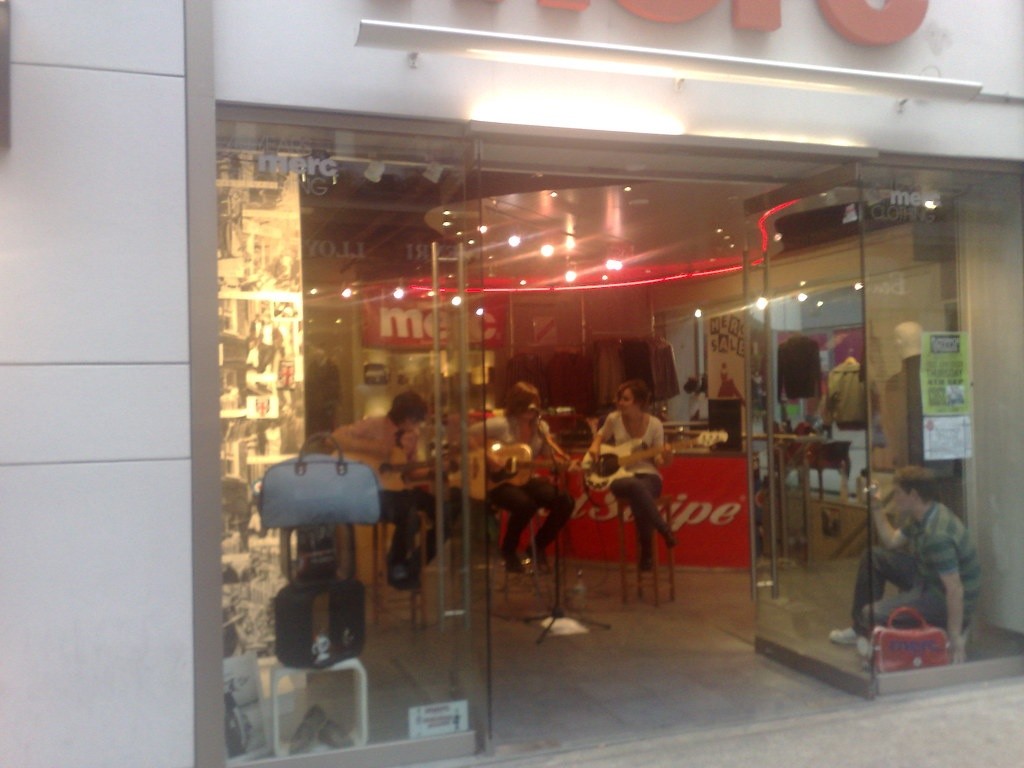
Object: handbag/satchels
223 610 269 762
272 551 365 668
253 430 382 529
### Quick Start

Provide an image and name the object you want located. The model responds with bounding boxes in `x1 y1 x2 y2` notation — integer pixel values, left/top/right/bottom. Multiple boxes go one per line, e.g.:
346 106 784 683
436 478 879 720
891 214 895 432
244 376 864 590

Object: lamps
474 306 483 315
451 294 462 307
392 283 407 299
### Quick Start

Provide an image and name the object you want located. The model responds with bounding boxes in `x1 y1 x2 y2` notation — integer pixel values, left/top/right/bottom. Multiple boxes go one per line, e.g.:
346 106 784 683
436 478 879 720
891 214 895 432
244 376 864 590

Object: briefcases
869 606 954 673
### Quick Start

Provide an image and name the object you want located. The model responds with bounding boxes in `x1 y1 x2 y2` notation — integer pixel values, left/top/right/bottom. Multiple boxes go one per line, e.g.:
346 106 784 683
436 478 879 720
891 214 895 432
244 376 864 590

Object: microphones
528 403 541 419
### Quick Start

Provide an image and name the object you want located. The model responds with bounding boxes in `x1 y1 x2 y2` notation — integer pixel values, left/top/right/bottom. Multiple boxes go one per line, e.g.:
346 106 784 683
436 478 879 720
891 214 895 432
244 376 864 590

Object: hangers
847 350 853 359
647 328 661 341
791 327 803 340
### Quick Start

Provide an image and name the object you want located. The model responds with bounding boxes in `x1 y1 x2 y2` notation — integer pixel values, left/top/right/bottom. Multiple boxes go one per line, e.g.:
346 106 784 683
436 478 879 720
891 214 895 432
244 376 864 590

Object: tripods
523 418 609 645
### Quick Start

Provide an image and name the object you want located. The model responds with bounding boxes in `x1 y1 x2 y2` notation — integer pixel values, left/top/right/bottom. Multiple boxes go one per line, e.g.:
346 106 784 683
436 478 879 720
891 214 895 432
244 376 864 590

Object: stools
372 510 455 630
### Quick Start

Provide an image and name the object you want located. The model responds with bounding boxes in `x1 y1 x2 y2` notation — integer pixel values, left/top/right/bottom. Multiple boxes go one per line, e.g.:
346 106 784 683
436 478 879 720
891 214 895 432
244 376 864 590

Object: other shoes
661 529 678 547
526 547 547 564
830 625 862 645
385 555 421 591
501 546 523 573
857 636 872 655
640 554 653 569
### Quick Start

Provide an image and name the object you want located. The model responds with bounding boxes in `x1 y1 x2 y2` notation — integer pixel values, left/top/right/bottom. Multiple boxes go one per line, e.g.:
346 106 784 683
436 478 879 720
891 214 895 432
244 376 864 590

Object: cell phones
863 485 877 504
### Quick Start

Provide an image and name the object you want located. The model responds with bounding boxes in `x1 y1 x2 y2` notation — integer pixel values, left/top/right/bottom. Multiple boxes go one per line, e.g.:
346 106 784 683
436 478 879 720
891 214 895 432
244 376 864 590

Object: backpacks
776 333 823 403
828 356 866 430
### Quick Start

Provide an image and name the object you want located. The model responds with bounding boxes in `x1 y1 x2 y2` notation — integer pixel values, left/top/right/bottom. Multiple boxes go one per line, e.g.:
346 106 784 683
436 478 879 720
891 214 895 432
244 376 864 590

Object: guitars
458 438 577 494
332 447 457 490
581 429 727 494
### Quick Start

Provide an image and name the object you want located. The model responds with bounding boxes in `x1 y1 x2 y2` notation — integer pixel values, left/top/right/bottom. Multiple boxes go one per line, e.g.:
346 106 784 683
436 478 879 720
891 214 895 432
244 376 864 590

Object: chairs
616 444 676 609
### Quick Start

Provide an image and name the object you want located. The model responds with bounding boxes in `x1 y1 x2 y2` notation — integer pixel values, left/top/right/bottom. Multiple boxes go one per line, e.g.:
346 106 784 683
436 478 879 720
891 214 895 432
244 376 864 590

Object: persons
465 381 577 575
324 389 458 590
580 379 680 571
829 464 983 664
884 320 968 527
